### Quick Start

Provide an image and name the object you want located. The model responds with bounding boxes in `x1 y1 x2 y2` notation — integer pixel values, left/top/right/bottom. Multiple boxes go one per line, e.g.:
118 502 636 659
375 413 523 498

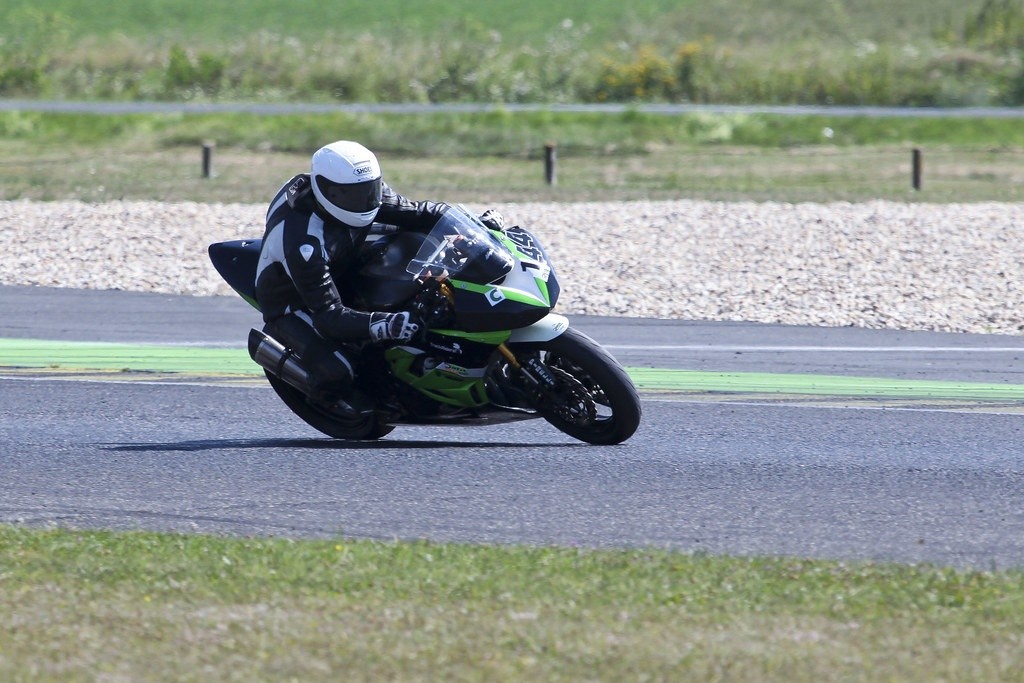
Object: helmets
309 140 384 227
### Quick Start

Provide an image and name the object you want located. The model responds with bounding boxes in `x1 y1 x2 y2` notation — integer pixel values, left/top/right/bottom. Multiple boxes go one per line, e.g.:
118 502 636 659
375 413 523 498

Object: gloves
369 311 419 345
477 209 504 231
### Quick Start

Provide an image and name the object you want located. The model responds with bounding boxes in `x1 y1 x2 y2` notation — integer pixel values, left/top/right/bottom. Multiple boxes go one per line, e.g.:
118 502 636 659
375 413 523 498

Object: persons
255 140 504 424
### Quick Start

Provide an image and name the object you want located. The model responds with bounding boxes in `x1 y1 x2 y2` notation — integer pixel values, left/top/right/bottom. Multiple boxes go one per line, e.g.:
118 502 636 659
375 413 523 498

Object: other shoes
304 382 364 425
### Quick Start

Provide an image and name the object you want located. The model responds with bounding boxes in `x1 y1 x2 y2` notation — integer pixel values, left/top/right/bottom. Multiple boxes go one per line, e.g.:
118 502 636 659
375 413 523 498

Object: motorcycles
207 203 643 446
